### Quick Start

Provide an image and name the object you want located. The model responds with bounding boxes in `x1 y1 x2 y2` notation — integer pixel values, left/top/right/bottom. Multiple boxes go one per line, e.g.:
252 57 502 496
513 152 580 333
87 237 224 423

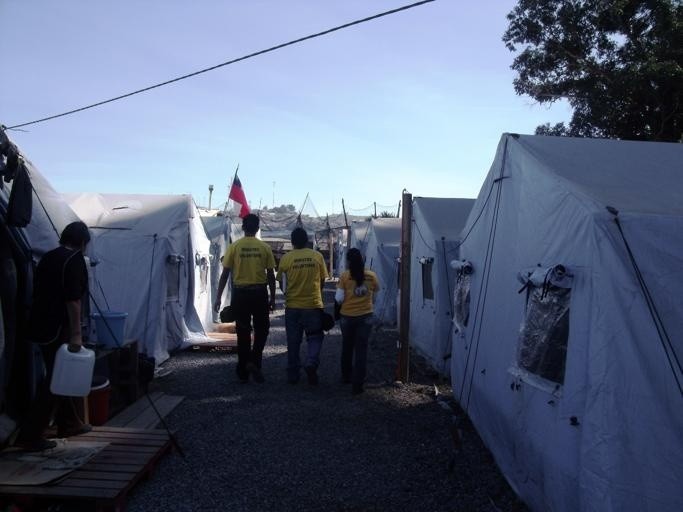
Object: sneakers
59 423 92 438
239 363 264 384
306 366 318 387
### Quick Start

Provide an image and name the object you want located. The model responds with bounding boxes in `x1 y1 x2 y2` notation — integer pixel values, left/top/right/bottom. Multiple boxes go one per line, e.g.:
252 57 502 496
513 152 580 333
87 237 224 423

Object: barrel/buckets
88 374 110 424
50 343 94 398
92 308 129 343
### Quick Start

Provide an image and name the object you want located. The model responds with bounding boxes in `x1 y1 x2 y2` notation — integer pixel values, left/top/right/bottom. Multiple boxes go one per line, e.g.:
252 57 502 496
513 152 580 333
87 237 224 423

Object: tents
1 126 264 455
338 133 683 512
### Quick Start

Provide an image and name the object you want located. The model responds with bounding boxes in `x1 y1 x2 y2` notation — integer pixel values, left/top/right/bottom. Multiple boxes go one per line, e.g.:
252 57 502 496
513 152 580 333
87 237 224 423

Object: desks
80 339 139 425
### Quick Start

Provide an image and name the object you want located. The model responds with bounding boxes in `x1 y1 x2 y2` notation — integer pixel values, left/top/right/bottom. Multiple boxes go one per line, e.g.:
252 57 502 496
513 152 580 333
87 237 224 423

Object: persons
213 213 277 385
277 226 329 386
333 247 380 394
